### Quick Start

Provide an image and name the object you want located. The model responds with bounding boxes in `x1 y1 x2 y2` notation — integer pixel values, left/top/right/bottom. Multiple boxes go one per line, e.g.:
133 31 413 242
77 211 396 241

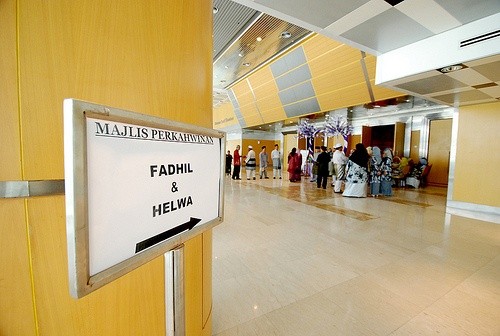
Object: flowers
322 114 354 138
297 118 319 138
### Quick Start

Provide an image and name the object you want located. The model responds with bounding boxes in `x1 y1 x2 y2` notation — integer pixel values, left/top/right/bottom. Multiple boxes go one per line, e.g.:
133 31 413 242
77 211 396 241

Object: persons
270 144 282 179
225 150 233 175
259 145 269 179
287 147 298 182
295 148 302 181
316 146 331 189
232 145 244 180
326 143 430 198
243 145 256 180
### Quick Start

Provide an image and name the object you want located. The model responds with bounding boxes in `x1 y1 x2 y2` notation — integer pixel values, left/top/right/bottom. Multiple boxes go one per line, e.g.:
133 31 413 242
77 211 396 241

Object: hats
248 145 253 149
334 143 341 148
